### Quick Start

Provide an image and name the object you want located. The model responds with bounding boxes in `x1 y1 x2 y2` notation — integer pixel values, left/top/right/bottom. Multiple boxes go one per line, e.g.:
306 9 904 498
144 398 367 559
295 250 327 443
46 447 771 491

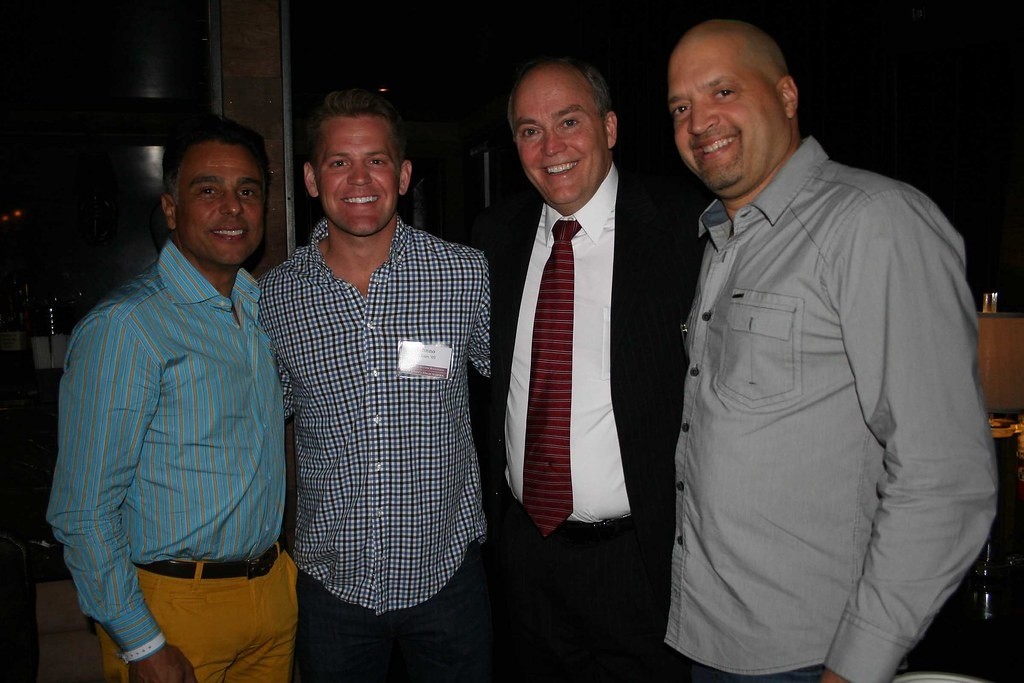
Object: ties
522 219 582 538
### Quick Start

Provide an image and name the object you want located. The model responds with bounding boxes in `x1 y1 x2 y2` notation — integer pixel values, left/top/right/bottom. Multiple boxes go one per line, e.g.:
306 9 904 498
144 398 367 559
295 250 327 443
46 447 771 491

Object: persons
473 50 695 683
46 115 301 683
258 92 492 683
665 19 999 683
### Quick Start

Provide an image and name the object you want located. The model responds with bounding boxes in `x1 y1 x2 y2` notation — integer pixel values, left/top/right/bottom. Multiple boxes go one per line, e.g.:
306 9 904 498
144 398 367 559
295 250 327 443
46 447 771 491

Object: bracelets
122 632 166 664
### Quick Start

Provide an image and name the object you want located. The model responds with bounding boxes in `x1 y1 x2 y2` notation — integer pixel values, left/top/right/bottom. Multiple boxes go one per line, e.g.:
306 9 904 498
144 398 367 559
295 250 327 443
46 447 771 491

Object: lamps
968 311 1024 578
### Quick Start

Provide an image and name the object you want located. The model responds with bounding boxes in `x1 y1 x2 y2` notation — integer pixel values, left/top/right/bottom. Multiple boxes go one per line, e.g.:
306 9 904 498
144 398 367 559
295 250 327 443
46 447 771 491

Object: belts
135 532 286 581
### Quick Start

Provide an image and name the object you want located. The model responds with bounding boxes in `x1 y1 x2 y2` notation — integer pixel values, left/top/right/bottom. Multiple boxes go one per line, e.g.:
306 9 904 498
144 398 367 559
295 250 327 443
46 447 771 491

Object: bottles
0 269 29 386
